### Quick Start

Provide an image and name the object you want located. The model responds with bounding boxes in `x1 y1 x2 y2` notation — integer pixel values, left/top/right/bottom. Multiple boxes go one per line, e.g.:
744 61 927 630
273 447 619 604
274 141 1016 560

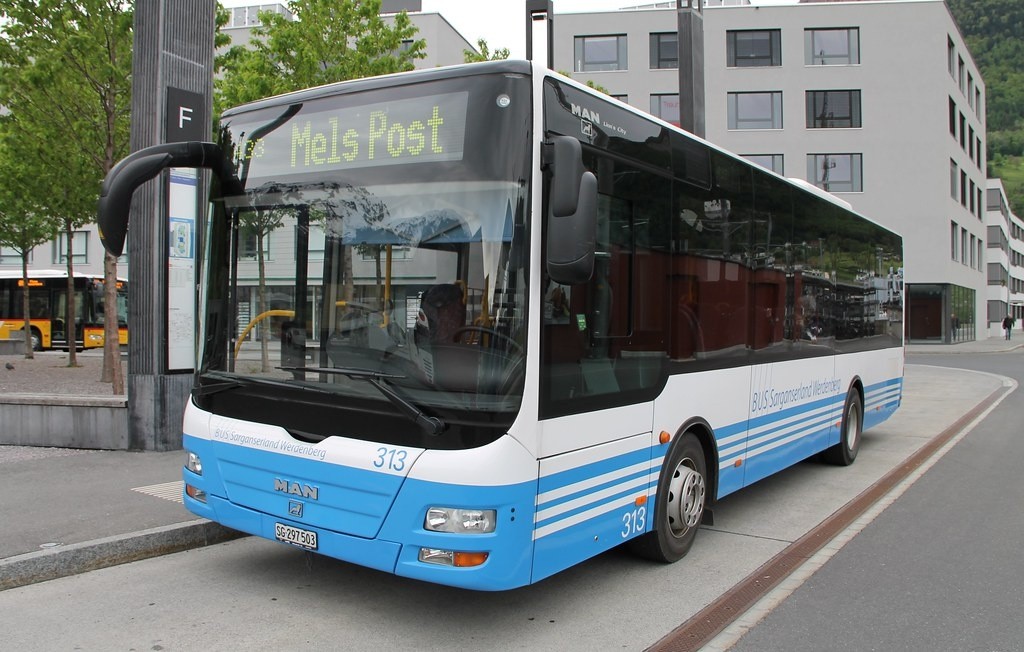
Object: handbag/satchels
1002 320 1006 329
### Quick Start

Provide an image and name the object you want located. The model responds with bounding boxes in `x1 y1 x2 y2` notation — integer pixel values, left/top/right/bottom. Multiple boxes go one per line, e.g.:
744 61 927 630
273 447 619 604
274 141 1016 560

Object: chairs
416 282 464 347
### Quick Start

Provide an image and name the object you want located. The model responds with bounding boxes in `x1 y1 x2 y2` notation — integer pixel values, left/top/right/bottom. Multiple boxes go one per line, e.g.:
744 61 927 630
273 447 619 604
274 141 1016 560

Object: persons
951 313 961 341
1003 314 1015 340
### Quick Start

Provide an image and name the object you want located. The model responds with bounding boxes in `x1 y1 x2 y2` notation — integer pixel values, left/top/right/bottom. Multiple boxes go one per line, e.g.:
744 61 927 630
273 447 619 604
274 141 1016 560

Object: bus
96 58 907 590
0 270 129 353
